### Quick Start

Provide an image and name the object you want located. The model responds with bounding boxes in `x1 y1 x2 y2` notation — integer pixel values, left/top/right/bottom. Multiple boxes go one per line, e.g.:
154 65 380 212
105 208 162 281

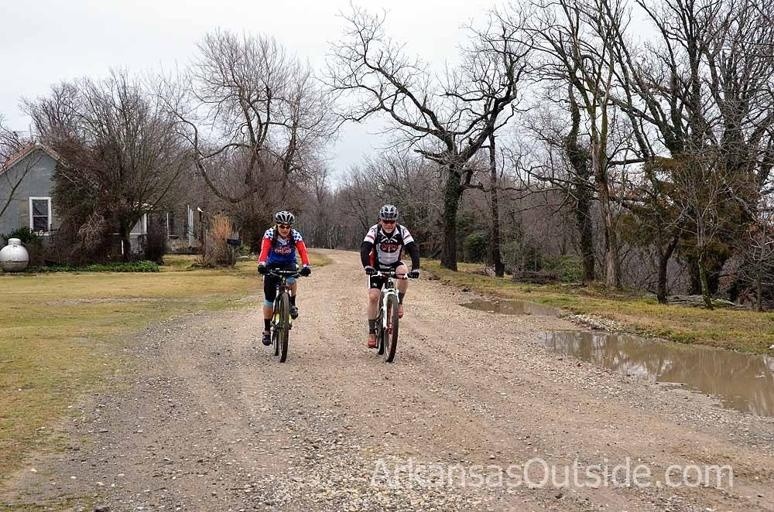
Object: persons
360 203 421 346
257 210 311 346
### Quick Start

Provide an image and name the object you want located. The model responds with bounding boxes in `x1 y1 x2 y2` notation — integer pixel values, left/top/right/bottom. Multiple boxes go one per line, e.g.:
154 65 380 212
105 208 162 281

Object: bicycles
370 267 410 362
265 270 301 364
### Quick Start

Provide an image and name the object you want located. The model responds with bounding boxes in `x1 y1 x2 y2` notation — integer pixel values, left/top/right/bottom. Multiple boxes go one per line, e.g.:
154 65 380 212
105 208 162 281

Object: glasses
382 219 395 224
279 225 292 229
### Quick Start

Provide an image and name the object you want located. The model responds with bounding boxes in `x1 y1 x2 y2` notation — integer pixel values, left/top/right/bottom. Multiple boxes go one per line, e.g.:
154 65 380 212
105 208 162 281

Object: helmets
275 210 295 224
377 204 400 221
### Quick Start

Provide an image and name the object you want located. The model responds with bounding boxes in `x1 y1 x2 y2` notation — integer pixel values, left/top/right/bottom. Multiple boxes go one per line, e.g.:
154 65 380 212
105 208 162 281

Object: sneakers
399 304 404 318
290 305 297 320
262 331 271 345
368 333 376 346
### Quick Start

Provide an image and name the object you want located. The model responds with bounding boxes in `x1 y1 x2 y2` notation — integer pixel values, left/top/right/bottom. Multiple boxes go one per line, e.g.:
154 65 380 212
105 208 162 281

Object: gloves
300 268 310 277
408 272 419 279
365 268 376 275
257 264 266 273
